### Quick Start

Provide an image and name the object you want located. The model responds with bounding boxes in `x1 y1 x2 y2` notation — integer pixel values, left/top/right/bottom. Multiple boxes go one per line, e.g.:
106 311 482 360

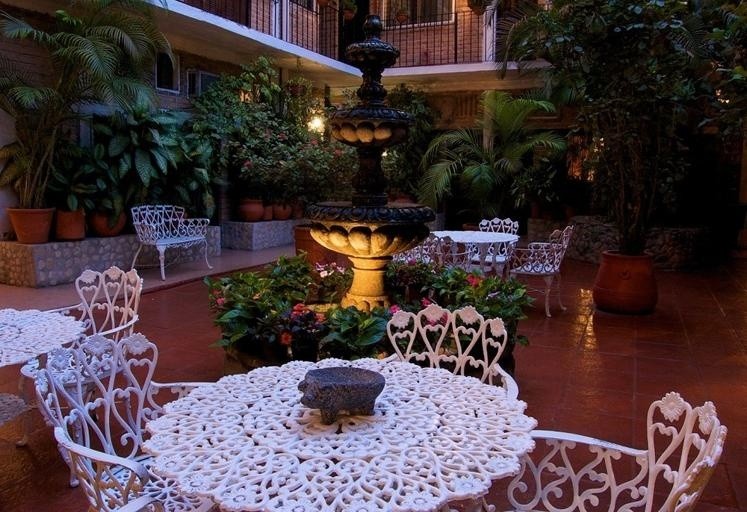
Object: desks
141 357 538 512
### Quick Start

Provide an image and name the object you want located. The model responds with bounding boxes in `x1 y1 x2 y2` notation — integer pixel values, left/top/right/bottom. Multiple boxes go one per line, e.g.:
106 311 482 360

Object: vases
239 199 303 222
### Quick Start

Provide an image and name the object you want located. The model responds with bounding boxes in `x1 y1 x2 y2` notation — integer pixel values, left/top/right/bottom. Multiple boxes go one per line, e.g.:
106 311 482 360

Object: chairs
1 266 143 487
34 333 216 512
386 305 518 397
393 218 577 320
482 392 729 512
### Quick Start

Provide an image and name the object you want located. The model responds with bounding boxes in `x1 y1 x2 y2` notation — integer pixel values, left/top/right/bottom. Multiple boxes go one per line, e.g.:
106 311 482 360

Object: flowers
205 252 387 377
388 260 536 364
188 56 361 211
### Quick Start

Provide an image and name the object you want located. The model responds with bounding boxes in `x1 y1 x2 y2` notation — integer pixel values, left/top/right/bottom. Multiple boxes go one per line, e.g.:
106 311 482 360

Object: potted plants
1 3 218 244
408 90 567 254
377 83 443 203
482 0 747 313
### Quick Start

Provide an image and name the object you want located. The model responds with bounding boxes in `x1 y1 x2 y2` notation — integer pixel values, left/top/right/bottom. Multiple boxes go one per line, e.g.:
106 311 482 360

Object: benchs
129 205 213 281
